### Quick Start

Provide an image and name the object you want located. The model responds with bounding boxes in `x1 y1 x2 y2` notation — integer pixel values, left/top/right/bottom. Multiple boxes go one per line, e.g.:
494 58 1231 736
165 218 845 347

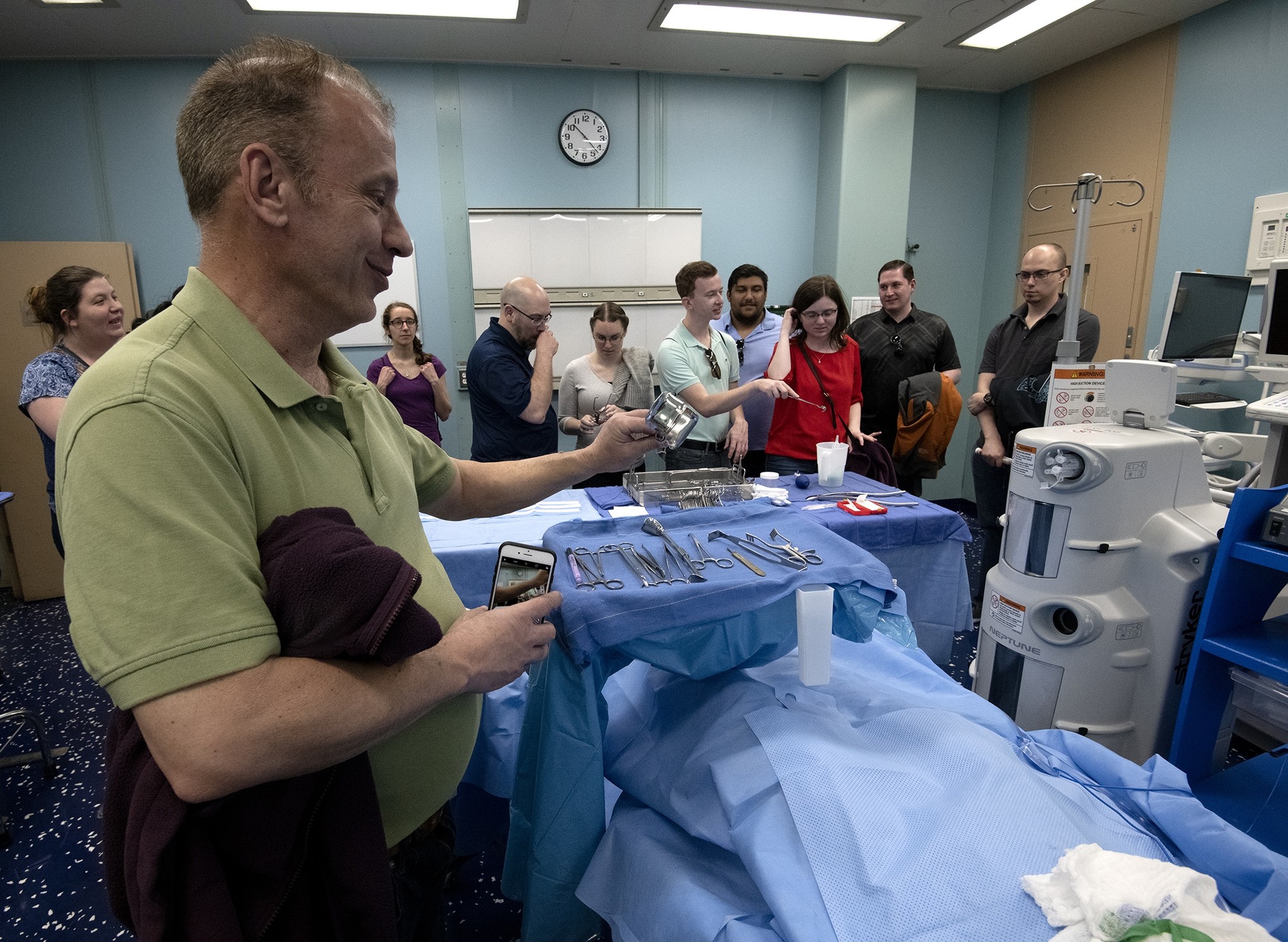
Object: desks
419 471 975 781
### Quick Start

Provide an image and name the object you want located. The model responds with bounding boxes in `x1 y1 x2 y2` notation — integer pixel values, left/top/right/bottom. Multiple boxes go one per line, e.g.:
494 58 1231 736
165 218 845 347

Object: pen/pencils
566 546 583 583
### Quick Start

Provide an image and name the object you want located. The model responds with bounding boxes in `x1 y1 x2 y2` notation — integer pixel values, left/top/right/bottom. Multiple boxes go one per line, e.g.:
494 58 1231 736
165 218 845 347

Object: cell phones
489 540 556 624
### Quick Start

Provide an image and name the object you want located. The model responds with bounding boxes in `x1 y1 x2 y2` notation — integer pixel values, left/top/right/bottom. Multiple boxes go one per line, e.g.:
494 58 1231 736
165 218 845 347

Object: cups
666 407 699 450
816 442 850 487
645 391 688 442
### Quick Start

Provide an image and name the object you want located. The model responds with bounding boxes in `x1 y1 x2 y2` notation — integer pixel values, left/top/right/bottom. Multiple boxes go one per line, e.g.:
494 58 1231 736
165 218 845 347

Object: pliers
806 490 919 506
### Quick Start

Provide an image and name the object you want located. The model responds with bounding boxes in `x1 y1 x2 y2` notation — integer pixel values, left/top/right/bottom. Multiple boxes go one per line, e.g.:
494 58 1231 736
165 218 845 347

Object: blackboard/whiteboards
325 242 425 349
474 303 688 392
468 206 703 290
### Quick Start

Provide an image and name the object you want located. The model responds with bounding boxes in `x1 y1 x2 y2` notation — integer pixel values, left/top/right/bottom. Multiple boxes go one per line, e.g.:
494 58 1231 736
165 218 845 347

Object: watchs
984 393 994 406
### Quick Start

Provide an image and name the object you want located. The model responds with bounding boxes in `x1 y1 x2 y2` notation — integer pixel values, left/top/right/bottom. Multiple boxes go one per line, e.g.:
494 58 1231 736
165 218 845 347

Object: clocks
558 109 610 166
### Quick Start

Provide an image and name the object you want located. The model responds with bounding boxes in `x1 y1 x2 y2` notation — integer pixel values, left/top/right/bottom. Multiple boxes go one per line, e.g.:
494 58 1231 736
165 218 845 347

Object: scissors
688 532 734 570
573 545 613 582
605 542 651 589
628 545 687 591
745 527 824 567
678 487 725 510
572 550 624 593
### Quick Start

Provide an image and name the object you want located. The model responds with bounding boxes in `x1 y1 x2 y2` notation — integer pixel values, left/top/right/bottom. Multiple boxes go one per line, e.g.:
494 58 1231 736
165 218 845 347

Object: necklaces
392 349 415 376
804 340 833 363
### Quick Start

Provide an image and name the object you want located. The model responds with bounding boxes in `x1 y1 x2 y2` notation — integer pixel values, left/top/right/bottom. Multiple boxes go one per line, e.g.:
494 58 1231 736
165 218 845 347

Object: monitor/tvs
1157 272 1253 361
1257 259 1288 365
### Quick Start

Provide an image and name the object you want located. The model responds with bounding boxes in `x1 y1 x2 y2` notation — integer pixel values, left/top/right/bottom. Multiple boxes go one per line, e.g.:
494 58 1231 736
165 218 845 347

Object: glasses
801 308 839 319
593 329 626 343
890 333 904 360
390 320 416 329
593 393 619 427
736 338 745 366
504 303 553 326
1016 269 1061 282
705 348 721 380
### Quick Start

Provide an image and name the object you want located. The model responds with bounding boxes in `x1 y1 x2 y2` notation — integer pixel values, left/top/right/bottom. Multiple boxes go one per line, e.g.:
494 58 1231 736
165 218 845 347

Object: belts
680 437 727 452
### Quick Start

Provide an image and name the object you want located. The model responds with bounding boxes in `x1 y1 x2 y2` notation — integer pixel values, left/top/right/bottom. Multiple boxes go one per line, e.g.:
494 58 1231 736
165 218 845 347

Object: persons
18 266 124 559
656 260 800 481
763 274 882 476
967 243 1099 623
558 302 654 489
848 259 961 496
471 276 558 462
710 263 803 478
55 37 675 942
366 302 452 448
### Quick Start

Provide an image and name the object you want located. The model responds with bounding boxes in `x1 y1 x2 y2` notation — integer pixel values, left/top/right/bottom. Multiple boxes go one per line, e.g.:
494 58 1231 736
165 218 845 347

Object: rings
420 365 423 368
